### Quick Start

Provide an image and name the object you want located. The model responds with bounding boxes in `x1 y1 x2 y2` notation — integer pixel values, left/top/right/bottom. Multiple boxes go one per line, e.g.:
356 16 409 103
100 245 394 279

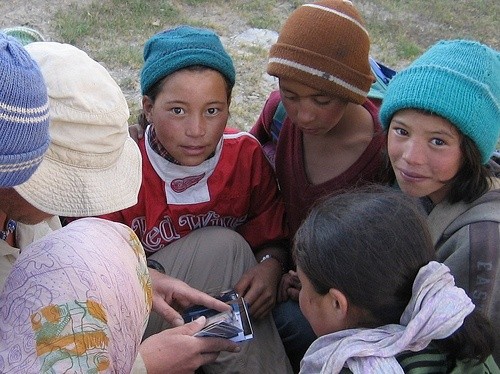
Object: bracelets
259 254 285 275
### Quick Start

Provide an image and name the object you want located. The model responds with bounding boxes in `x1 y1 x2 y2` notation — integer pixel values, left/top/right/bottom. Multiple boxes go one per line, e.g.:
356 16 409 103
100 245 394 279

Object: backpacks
269 57 398 147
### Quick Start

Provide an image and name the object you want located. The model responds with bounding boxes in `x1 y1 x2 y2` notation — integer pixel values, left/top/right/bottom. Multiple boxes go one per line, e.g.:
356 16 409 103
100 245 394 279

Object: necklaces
0 219 16 239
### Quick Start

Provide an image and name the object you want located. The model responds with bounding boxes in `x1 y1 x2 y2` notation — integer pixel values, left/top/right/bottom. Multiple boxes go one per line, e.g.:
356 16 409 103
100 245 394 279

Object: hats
379 39 500 165
0 33 50 187
140 25 235 95
267 0 375 105
12 42 143 217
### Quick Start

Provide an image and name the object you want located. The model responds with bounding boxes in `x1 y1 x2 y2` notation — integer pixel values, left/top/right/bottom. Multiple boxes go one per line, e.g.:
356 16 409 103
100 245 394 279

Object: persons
0 31 294 374
64 25 292 322
247 0 398 374
295 184 500 374
377 39 500 374
